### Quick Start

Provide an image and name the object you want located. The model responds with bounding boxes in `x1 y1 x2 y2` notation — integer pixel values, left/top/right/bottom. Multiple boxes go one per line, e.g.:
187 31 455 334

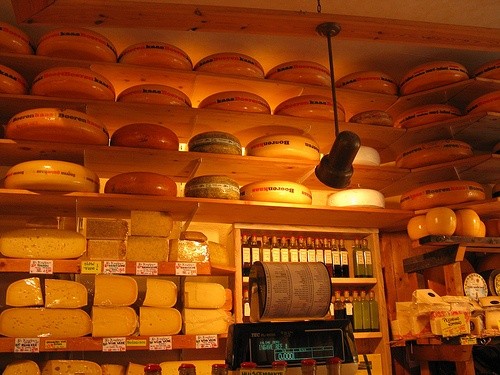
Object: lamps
314 20 362 188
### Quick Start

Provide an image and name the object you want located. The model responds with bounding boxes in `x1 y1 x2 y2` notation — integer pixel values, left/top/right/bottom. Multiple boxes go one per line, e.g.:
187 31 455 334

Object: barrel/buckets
249 260 331 319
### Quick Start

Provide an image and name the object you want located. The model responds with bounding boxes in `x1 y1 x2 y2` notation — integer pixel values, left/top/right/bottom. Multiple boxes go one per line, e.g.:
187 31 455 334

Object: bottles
335 290 345 320
325 357 342 375
361 239 373 278
178 363 196 375
367 289 379 332
352 290 363 332
272 361 288 375
211 363 229 375
359 290 371 332
301 360 317 375
242 290 250 323
329 301 335 320
342 290 354 332
144 364 162 375
240 231 349 278
240 362 257 375
353 238 365 278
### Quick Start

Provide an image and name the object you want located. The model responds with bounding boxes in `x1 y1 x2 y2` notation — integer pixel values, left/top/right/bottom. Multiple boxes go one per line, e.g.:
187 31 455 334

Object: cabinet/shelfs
0 51 500 375
